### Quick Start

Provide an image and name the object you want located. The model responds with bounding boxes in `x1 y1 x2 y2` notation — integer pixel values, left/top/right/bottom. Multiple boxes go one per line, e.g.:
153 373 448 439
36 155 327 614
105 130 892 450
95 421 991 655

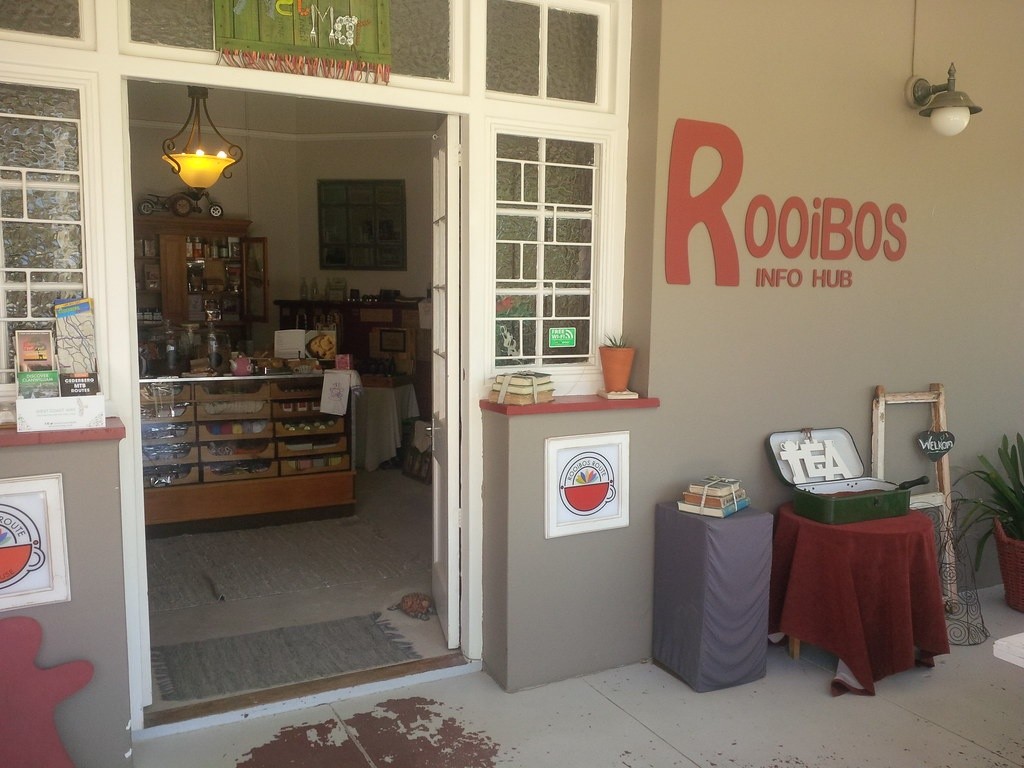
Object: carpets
150 610 423 701
145 513 433 616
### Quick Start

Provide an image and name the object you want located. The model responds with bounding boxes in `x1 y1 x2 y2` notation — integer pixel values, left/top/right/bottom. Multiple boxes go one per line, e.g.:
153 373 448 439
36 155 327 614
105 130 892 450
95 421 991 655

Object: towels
320 369 363 415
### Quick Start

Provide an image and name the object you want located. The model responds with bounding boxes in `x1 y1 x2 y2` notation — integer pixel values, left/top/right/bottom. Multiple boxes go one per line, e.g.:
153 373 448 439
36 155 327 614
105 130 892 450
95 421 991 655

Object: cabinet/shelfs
133 215 270 352
138 373 357 540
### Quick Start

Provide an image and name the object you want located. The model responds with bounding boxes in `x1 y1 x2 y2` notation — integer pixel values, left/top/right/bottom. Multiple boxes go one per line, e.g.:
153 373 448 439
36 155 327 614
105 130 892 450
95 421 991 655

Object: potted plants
951 431 1024 613
599 332 636 393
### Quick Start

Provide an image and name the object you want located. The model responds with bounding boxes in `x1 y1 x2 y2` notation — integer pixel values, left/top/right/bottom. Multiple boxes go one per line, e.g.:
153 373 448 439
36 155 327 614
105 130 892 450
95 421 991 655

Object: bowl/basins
250 358 287 373
288 358 317 373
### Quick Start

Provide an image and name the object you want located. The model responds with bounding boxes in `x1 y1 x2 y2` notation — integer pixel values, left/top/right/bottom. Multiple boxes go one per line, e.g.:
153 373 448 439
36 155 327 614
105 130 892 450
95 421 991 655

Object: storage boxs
766 427 912 525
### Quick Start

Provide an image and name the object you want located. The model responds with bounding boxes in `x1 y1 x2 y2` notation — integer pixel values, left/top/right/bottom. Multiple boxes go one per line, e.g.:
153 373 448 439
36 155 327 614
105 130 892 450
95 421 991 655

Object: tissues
326 269 346 290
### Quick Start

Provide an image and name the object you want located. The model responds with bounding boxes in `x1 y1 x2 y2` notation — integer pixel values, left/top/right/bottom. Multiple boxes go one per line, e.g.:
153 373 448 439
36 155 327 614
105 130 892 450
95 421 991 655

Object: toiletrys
185 237 228 258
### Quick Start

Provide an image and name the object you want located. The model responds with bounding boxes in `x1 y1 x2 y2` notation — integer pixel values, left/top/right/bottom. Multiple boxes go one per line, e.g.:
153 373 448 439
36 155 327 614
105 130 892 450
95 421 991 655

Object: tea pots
229 356 254 376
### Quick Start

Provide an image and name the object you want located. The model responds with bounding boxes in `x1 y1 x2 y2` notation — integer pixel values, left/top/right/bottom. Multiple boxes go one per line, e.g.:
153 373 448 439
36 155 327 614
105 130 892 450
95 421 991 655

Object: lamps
905 61 983 136
161 86 243 198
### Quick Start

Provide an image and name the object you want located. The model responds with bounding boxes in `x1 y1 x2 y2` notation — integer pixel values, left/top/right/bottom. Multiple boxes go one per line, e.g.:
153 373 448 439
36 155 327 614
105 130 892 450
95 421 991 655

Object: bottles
301 277 308 299
310 277 319 298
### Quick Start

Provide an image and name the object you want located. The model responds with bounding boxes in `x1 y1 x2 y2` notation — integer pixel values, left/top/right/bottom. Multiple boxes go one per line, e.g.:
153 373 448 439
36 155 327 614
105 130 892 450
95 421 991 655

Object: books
599 388 638 399
489 370 556 405
677 476 751 518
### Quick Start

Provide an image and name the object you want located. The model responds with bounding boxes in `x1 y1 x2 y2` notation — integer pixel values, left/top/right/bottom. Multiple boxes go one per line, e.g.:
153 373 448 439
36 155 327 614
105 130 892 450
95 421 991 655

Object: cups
350 289 359 299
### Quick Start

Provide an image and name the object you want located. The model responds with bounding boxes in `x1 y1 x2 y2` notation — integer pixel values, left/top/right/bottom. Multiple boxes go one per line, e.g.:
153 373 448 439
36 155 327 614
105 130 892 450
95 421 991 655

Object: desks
651 500 773 693
768 500 950 697
357 383 421 473
